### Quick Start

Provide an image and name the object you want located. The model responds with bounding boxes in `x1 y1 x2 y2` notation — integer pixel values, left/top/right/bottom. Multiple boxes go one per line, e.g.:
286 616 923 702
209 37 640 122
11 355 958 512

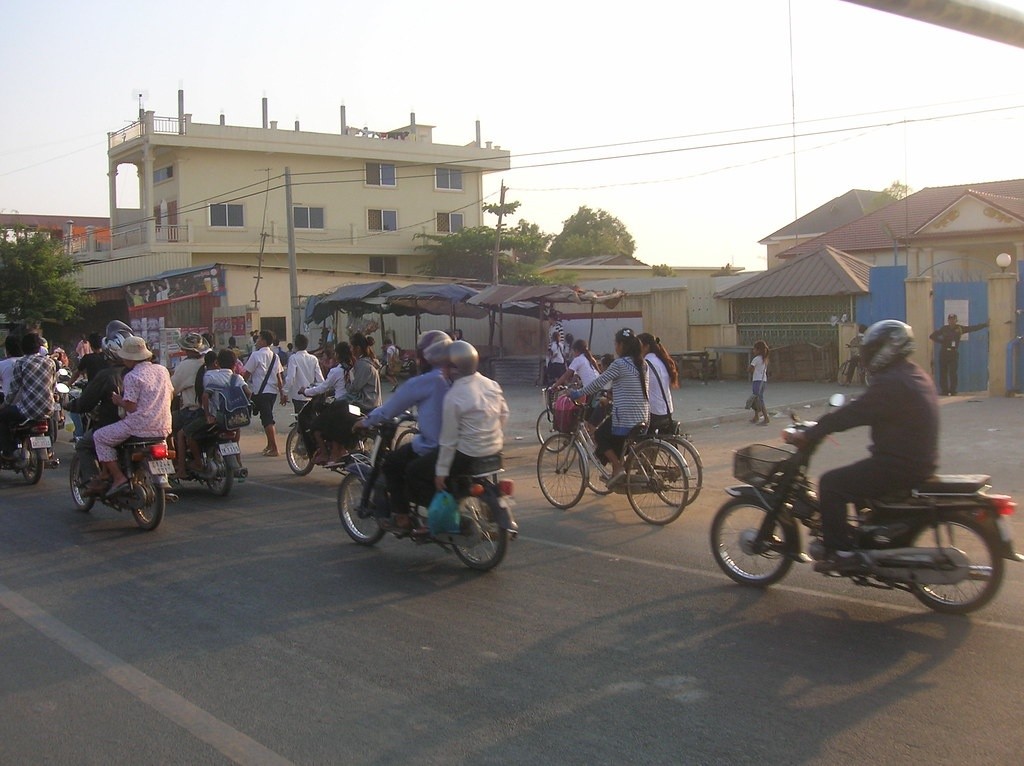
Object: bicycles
534 382 706 526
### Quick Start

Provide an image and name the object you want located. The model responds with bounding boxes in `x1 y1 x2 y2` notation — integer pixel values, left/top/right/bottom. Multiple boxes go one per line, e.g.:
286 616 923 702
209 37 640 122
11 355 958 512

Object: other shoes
606 471 626 490
814 551 862 573
0 434 349 497
379 513 412 533
599 473 612 482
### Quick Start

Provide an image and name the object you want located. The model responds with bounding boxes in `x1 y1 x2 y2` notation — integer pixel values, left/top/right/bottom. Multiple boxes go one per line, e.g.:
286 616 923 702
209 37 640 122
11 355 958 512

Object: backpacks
554 394 579 432
206 373 250 430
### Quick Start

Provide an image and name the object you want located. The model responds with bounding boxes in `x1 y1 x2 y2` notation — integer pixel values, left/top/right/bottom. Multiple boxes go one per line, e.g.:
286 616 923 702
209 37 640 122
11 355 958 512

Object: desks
670 350 709 383
705 345 784 383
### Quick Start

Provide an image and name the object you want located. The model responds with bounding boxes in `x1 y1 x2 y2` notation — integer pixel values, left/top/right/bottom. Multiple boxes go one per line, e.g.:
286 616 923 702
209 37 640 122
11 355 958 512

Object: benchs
682 353 721 379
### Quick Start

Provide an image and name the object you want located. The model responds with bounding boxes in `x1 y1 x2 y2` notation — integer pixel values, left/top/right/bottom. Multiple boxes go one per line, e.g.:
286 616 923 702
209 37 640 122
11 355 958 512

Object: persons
544 313 575 386
846 324 867 385
127 279 184 307
552 327 679 487
929 313 989 396
747 341 770 423
353 328 510 537
0 321 381 496
801 319 938 560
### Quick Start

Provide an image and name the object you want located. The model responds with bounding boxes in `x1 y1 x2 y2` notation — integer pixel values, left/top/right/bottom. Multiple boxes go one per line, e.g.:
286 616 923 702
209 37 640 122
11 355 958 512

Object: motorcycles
166 425 250 498
54 382 180 530
709 392 1023 615
0 417 59 485
337 405 520 571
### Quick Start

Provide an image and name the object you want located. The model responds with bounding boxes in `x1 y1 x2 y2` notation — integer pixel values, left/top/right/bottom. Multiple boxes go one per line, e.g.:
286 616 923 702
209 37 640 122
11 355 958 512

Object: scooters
283 380 382 478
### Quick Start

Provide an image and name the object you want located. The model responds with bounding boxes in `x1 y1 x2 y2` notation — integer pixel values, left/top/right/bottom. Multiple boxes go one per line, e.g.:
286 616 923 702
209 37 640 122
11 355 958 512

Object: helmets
417 329 452 364
860 319 917 370
102 329 133 355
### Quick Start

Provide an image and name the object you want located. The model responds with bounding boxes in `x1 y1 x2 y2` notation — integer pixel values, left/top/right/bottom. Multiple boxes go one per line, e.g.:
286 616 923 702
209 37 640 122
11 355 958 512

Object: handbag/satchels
427 488 460 538
546 382 566 408
745 394 762 413
249 392 261 416
668 420 681 435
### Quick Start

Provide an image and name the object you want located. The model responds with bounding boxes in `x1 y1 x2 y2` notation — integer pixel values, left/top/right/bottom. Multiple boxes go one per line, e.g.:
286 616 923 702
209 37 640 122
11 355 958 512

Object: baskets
733 443 795 487
543 385 568 409
551 408 585 435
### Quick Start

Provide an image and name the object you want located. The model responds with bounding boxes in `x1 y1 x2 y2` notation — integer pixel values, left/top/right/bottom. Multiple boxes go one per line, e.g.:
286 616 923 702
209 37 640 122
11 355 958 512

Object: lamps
996 253 1012 273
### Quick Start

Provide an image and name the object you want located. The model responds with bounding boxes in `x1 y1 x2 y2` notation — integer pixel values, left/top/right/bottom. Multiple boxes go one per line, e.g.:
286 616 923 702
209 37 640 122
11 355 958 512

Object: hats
948 314 958 320
176 332 210 352
444 340 479 377
118 336 152 360
